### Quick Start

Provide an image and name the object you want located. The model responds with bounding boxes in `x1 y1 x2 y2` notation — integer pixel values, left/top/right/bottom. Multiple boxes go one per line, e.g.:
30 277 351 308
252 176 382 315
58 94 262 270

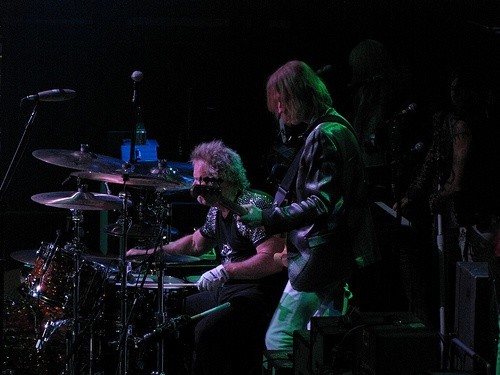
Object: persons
393 98 500 255
237 61 372 375
127 140 284 375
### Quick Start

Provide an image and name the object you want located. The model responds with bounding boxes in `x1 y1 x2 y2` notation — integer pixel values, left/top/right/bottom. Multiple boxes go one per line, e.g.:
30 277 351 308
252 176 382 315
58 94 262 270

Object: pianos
374 199 414 232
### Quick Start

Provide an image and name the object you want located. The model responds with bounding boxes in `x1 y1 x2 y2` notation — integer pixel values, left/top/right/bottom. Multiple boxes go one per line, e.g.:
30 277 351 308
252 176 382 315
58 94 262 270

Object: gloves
197 264 230 291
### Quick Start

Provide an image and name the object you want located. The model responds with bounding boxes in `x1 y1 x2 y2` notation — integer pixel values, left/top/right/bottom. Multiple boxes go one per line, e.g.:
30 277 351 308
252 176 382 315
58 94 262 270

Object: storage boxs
453 262 500 375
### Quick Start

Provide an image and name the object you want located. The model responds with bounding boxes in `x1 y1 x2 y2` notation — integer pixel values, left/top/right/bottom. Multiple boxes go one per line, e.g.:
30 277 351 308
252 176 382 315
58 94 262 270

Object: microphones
386 103 416 125
26 89 76 101
131 71 143 82
390 142 424 164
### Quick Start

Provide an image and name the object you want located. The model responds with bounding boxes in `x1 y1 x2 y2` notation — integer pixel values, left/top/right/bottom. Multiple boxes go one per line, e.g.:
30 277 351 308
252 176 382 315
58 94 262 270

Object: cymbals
70 170 176 187
31 147 123 173
31 190 132 211
11 249 38 265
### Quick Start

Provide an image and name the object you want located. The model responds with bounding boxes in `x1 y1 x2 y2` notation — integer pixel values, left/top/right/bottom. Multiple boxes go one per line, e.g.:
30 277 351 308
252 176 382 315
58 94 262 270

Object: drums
22 239 189 318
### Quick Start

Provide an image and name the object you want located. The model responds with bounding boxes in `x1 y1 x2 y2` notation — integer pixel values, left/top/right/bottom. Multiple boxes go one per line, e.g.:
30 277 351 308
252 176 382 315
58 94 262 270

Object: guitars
190 176 248 218
